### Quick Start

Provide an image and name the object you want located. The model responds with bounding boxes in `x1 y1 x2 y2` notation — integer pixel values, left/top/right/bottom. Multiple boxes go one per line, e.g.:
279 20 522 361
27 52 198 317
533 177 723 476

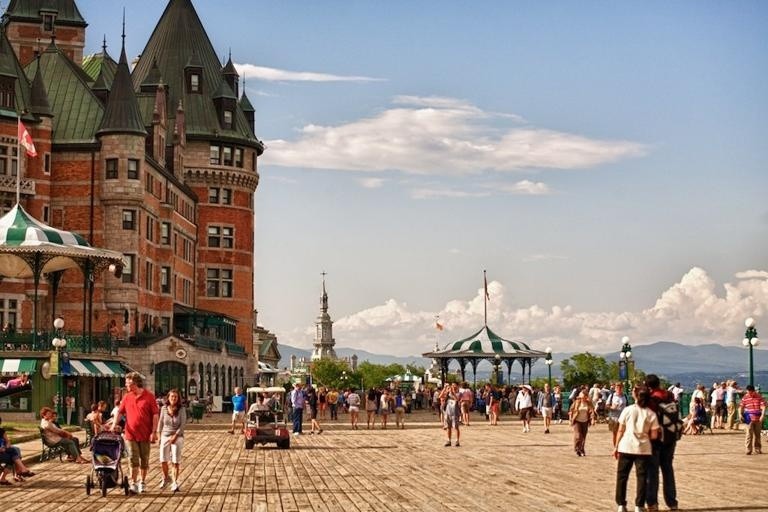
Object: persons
614 383 659 512
645 374 678 510
205 391 215 416
0 322 198 494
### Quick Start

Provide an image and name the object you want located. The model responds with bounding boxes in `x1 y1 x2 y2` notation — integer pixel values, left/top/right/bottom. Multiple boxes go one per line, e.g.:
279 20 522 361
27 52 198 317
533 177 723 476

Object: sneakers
634 505 647 512
171 481 180 492
17 470 36 478
160 475 171 489
310 428 322 435
523 427 549 434
129 483 137 495
139 481 148 494
444 442 460 447
1 478 13 486
618 504 629 512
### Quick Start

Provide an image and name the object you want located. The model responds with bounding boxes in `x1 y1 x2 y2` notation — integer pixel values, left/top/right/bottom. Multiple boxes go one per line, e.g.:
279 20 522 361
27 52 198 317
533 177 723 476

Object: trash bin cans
191 403 204 424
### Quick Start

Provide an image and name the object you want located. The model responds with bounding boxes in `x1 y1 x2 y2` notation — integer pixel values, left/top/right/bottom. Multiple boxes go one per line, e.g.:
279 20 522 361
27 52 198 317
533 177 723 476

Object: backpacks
648 392 682 443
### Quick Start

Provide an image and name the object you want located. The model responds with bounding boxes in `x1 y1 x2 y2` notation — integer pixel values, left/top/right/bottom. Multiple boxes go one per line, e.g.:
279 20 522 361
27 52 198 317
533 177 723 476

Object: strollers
83 428 129 498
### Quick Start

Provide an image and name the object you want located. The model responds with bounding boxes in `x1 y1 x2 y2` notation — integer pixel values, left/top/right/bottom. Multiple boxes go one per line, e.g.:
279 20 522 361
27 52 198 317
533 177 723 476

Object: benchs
36 423 74 464
696 413 714 438
0 454 25 480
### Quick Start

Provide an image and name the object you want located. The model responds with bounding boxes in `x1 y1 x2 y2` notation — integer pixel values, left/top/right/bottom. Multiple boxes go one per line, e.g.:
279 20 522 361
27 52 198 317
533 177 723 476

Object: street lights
740 319 760 391
340 371 348 387
492 354 502 385
620 334 634 397
544 347 553 389
50 316 67 425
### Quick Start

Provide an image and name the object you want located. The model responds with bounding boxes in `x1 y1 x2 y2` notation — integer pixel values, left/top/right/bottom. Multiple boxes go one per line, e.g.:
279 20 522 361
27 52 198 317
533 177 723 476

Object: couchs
83 418 96 451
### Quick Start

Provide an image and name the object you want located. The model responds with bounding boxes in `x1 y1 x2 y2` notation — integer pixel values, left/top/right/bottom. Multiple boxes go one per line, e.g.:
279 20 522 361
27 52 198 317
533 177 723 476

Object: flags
485 277 490 300
16 120 40 159
436 318 443 331
